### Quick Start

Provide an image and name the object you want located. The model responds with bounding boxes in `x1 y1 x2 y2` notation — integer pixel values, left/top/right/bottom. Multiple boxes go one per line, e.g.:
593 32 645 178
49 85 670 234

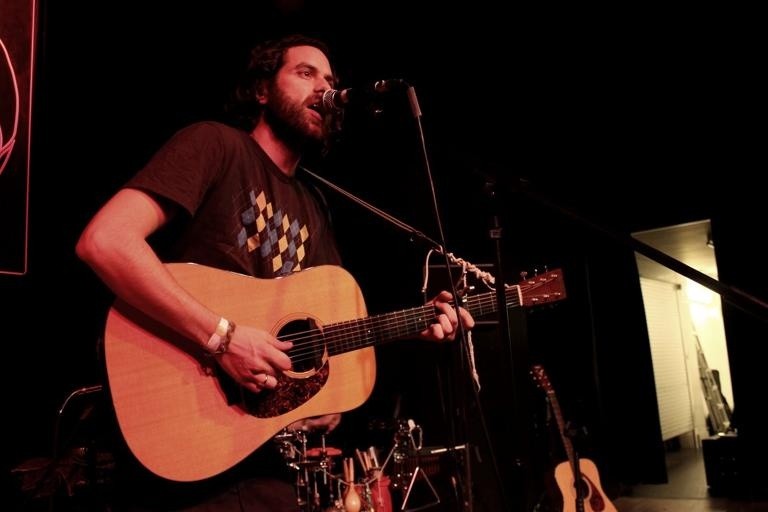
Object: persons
75 35 477 512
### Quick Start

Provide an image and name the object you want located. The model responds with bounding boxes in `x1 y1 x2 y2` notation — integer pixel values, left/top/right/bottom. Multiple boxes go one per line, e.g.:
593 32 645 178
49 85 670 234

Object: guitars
96 263 566 483
528 359 615 512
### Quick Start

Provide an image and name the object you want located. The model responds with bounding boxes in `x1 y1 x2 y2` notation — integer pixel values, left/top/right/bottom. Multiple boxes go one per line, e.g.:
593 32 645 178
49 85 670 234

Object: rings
262 374 268 384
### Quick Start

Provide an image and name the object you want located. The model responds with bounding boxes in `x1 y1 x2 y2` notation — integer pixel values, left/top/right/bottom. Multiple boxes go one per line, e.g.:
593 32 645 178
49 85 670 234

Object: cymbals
273 433 294 439
303 446 342 457
287 460 336 469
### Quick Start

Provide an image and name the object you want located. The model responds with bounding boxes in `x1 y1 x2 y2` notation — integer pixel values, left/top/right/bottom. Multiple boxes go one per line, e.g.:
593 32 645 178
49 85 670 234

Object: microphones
321 78 403 114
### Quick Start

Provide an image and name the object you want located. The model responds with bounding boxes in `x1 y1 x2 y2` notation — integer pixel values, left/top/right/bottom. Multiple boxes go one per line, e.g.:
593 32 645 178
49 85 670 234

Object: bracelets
205 316 236 357
